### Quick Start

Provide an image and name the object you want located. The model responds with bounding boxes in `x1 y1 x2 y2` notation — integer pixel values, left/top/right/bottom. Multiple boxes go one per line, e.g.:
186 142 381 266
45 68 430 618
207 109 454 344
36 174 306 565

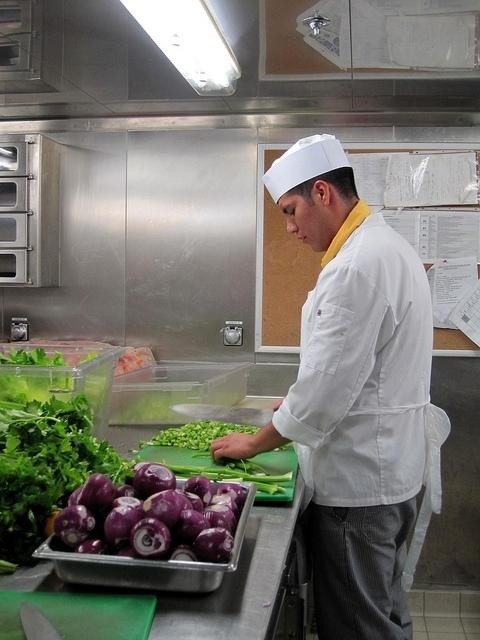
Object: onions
52 461 245 564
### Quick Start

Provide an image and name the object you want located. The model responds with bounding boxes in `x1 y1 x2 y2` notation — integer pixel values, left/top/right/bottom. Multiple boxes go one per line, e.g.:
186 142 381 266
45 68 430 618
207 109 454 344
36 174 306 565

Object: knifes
170 403 274 427
19 602 62 640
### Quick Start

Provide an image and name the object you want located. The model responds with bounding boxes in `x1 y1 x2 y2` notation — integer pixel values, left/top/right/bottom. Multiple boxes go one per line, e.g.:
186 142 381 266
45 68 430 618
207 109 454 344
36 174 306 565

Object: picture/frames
258 1 479 84
254 143 480 356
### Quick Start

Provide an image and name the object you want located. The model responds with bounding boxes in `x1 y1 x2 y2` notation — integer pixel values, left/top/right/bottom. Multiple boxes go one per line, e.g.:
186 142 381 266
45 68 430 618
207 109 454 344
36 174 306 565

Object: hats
260 130 354 206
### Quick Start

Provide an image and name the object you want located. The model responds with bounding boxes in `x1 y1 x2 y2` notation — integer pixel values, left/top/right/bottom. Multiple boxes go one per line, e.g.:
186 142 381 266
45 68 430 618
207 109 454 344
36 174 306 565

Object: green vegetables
138 465 295 495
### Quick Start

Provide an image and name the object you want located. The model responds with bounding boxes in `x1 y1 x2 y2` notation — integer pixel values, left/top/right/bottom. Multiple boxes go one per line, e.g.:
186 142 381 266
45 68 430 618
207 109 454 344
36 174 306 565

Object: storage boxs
0 340 127 413
107 362 251 423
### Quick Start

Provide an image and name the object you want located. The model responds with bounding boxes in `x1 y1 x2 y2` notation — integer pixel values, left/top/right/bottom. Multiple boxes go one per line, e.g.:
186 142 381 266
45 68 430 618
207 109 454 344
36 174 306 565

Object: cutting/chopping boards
134 442 299 502
0 589 156 638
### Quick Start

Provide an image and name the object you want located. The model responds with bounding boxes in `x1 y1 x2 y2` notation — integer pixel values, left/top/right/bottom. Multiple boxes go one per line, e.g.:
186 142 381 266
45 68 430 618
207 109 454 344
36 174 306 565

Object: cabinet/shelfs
0 0 66 93
0 134 61 286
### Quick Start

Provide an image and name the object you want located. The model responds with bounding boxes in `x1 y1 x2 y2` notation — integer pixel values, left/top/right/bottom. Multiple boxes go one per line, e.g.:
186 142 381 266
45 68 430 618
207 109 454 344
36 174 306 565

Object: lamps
119 0 242 99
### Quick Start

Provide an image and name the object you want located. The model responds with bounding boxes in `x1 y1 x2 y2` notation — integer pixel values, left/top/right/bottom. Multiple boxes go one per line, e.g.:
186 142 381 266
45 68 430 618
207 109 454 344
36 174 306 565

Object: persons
209 133 433 640
